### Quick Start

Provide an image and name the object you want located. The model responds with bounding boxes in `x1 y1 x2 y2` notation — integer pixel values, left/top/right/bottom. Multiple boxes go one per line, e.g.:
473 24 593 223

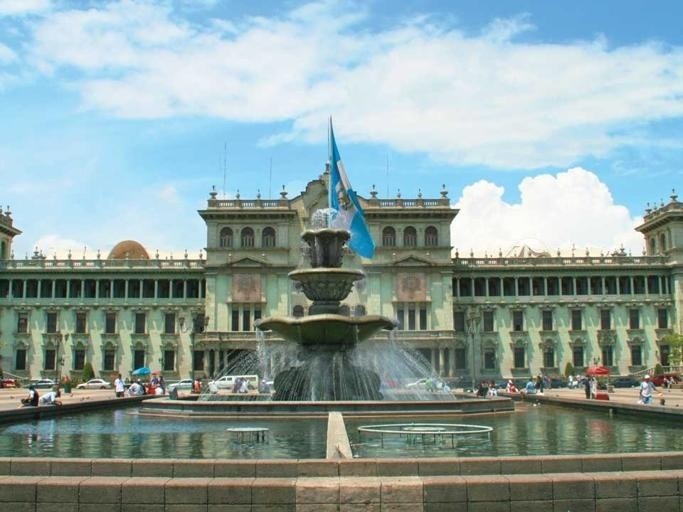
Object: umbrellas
149 370 160 378
131 368 151 383
585 365 608 375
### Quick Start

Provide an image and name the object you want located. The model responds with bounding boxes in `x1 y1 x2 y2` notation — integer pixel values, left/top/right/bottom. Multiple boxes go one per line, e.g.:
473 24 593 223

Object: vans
207 374 258 390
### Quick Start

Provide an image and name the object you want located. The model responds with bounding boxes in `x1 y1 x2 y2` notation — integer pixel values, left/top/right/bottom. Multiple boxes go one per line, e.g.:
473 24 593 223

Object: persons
17 370 270 408
16 371 272 410
380 371 682 409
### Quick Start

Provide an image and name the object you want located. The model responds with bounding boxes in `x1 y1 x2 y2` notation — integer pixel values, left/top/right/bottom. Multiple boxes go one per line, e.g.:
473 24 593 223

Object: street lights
177 310 209 392
466 311 481 390
596 329 616 391
53 338 59 383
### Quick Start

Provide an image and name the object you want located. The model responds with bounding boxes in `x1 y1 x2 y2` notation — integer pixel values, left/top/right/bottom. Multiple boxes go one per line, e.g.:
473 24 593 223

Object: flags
326 118 375 260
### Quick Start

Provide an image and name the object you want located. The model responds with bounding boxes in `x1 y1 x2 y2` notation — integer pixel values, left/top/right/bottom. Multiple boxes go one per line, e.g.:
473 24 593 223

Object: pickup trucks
0 377 17 389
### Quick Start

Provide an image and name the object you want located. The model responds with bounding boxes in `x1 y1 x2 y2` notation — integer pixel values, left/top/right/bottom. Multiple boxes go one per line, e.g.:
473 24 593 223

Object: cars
75 377 112 389
167 379 202 389
22 379 57 388
406 373 682 390
